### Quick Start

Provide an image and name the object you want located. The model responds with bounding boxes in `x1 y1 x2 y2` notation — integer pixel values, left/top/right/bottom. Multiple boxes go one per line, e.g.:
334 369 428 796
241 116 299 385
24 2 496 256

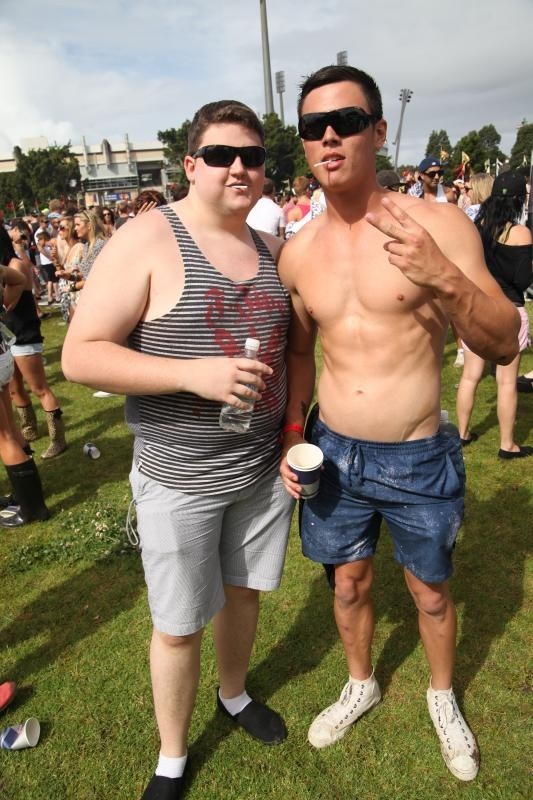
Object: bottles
219 337 262 433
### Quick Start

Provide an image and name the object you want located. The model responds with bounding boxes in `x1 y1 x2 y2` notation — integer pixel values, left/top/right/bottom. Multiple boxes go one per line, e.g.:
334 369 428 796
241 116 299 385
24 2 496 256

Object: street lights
393 88 414 170
275 70 286 126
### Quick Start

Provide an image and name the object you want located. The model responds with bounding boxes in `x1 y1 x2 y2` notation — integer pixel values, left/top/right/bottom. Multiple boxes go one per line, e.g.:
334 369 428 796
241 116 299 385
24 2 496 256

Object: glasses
42 220 49 224
103 213 111 217
55 221 60 223
298 105 375 142
424 170 443 179
190 143 267 167
463 186 473 192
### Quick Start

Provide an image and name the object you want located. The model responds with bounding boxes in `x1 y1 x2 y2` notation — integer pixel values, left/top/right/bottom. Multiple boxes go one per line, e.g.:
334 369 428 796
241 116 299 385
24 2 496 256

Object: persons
61 101 295 800
278 67 522 781
0 157 533 525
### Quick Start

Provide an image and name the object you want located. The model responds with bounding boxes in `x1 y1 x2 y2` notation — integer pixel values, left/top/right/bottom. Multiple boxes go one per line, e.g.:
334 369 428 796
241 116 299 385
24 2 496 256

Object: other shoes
461 431 477 447
216 684 287 745
142 746 190 800
498 446 533 459
0 681 16 710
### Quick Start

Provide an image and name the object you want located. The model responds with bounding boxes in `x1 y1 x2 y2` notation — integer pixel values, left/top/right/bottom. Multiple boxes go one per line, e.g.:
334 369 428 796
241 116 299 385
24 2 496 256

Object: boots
0 457 48 526
16 402 39 442
41 408 66 459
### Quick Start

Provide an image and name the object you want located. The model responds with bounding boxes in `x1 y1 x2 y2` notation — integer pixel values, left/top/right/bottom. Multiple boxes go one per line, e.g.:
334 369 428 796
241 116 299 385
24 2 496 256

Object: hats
420 159 443 174
377 170 406 189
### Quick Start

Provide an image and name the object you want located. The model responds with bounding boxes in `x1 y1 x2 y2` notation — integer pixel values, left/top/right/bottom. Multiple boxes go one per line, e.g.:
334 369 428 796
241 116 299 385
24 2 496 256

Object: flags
439 150 449 160
496 159 503 177
484 159 491 175
461 150 470 167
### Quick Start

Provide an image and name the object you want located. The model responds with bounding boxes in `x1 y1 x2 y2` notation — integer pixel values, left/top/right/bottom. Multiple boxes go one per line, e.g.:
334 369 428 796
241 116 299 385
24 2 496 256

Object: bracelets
283 424 303 433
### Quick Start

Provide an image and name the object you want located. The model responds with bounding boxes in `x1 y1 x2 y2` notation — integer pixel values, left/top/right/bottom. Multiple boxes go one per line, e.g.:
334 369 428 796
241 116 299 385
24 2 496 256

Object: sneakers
307 664 382 749
426 675 480 782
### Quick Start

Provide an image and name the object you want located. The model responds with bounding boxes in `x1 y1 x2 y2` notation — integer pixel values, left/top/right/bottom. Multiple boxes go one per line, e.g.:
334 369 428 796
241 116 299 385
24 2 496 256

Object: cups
287 442 324 502
0 718 40 749
83 443 101 459
